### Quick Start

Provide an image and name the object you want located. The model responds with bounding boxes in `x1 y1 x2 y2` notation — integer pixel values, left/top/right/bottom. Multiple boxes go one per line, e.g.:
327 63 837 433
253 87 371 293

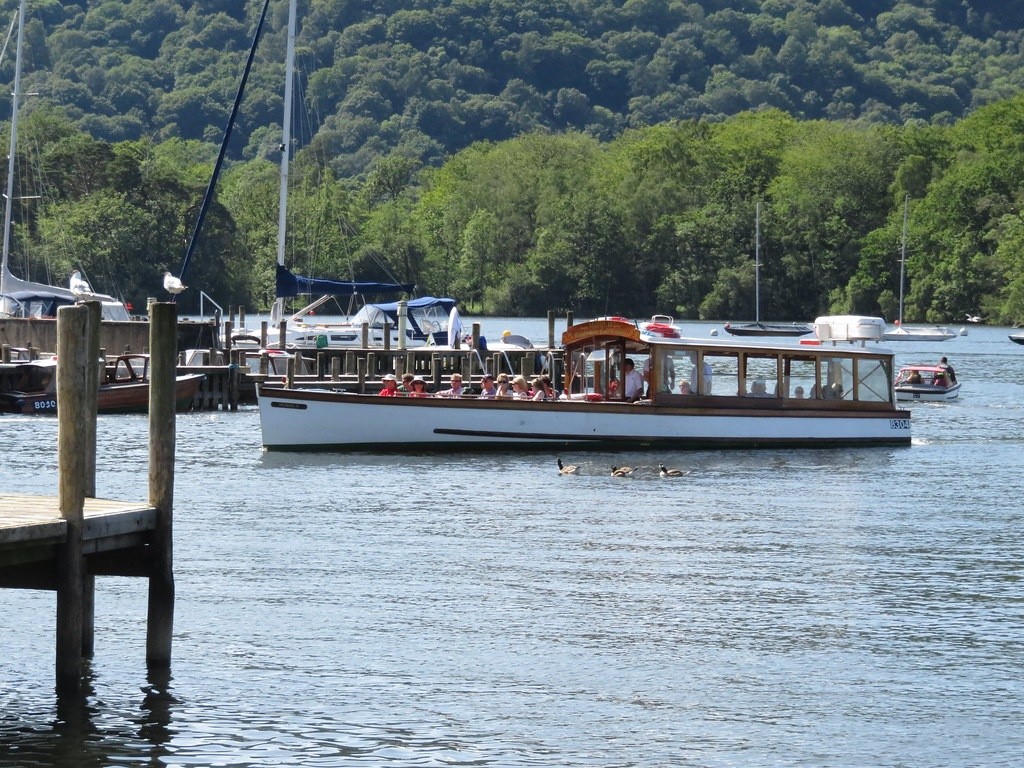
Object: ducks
658 463 696 476
556 458 583 474
608 465 642 476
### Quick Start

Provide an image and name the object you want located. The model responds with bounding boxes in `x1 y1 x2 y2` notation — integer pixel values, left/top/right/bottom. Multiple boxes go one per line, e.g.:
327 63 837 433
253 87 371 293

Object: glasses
679 384 685 388
482 380 490 382
415 382 423 385
512 384 516 386
451 380 460 382
403 381 408 384
498 381 508 384
796 390 804 394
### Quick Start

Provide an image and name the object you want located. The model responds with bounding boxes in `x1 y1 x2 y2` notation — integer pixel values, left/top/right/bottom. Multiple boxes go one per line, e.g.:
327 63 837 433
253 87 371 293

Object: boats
966 313 986 325
1008 334 1024 345
255 298 915 456
636 314 684 338
894 365 962 403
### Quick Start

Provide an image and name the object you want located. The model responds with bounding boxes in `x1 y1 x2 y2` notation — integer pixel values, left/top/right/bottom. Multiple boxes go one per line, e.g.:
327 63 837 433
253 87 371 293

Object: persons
678 353 712 395
397 373 414 396
795 383 843 400
531 375 552 400
933 357 956 382
406 376 429 397
378 374 402 397
625 358 643 403
512 378 527 400
478 376 495 398
643 347 675 398
751 380 768 396
496 374 513 397
435 373 463 397
462 334 486 350
907 370 924 385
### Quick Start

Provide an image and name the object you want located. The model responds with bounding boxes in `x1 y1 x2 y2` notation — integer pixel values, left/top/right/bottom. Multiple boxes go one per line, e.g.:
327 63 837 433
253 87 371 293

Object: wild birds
161 269 189 304
69 268 96 305
965 312 982 323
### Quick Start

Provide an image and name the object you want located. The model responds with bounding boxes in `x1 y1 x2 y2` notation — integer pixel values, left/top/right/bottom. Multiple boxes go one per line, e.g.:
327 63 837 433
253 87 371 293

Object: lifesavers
559 393 602 401
640 321 682 334
591 317 630 323
258 350 288 356
640 331 681 338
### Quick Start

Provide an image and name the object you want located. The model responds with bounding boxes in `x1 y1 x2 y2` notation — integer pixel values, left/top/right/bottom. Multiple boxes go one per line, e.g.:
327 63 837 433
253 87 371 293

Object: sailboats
881 194 959 342
723 201 815 337
0 0 549 420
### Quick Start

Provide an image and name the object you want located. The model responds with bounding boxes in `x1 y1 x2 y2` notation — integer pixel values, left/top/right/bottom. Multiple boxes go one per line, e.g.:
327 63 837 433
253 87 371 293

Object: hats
480 375 493 379
409 376 428 386
381 374 399 381
